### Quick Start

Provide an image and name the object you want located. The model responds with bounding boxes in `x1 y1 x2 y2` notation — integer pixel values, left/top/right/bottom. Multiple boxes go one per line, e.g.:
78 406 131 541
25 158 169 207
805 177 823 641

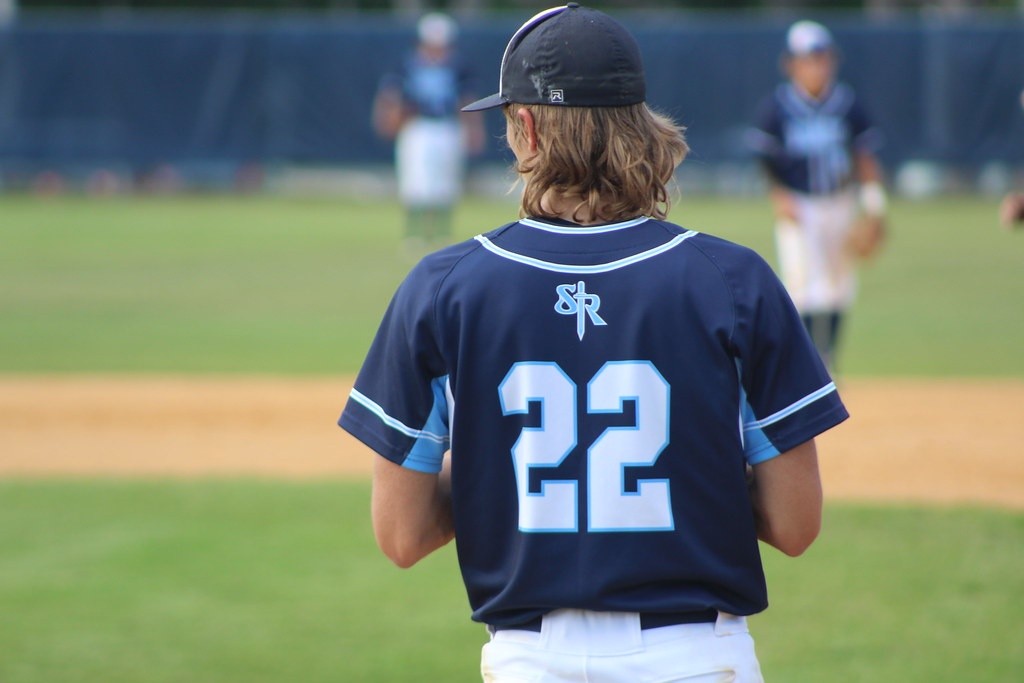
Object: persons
375 13 486 239
336 3 849 683
745 22 887 353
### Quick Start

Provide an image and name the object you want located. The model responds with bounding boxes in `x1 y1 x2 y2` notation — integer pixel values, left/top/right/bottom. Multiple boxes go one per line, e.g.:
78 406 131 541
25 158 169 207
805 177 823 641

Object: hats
461 3 646 112
787 21 834 56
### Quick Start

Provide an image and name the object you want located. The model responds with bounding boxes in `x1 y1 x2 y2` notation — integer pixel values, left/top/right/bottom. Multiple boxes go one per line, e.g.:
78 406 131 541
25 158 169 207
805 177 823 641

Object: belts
493 608 718 633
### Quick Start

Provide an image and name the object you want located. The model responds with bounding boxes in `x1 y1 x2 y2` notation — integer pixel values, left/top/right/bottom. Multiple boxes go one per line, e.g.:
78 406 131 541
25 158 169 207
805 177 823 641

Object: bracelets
860 182 886 217
